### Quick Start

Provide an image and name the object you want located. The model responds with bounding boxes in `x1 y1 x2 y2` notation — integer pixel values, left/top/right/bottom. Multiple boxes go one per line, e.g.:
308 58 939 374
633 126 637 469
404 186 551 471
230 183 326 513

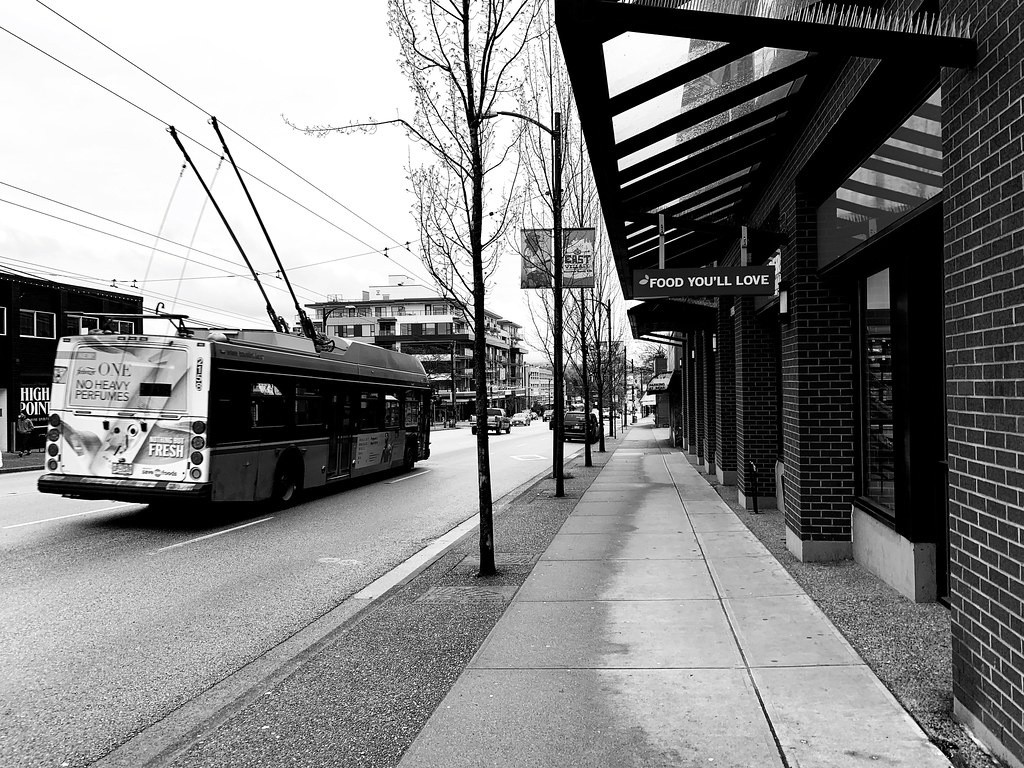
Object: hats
18 413 26 419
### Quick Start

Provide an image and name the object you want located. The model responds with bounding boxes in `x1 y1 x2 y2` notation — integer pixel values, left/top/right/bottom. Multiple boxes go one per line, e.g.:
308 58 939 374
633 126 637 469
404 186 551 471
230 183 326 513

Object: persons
16 410 34 458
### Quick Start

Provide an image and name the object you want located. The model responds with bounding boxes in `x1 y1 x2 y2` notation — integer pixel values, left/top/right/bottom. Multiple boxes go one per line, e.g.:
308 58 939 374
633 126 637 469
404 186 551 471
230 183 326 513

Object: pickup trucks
470 408 512 435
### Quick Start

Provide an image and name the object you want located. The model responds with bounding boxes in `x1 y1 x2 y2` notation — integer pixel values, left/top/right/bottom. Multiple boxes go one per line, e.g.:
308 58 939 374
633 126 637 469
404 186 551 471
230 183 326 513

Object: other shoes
19 453 23 457
26 452 31 455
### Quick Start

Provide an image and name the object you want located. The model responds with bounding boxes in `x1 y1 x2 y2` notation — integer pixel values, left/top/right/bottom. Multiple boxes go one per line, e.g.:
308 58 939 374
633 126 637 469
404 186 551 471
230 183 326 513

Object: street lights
575 297 614 438
323 306 356 333
474 110 564 501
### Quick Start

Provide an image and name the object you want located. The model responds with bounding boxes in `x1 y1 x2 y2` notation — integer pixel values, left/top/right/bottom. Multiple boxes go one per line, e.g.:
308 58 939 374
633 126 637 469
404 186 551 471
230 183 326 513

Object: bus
37 328 431 514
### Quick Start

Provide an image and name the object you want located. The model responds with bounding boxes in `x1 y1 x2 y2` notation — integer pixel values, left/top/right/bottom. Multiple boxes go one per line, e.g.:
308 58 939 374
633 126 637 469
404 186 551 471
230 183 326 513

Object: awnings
646 371 673 394
626 301 687 347
640 392 656 406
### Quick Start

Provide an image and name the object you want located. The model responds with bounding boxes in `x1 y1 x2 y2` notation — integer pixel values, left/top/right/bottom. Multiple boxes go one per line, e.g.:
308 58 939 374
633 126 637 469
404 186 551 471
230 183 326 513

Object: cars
548 411 555 430
532 412 538 421
510 413 531 426
525 413 533 421
563 411 605 443
601 408 621 422
542 410 554 422
